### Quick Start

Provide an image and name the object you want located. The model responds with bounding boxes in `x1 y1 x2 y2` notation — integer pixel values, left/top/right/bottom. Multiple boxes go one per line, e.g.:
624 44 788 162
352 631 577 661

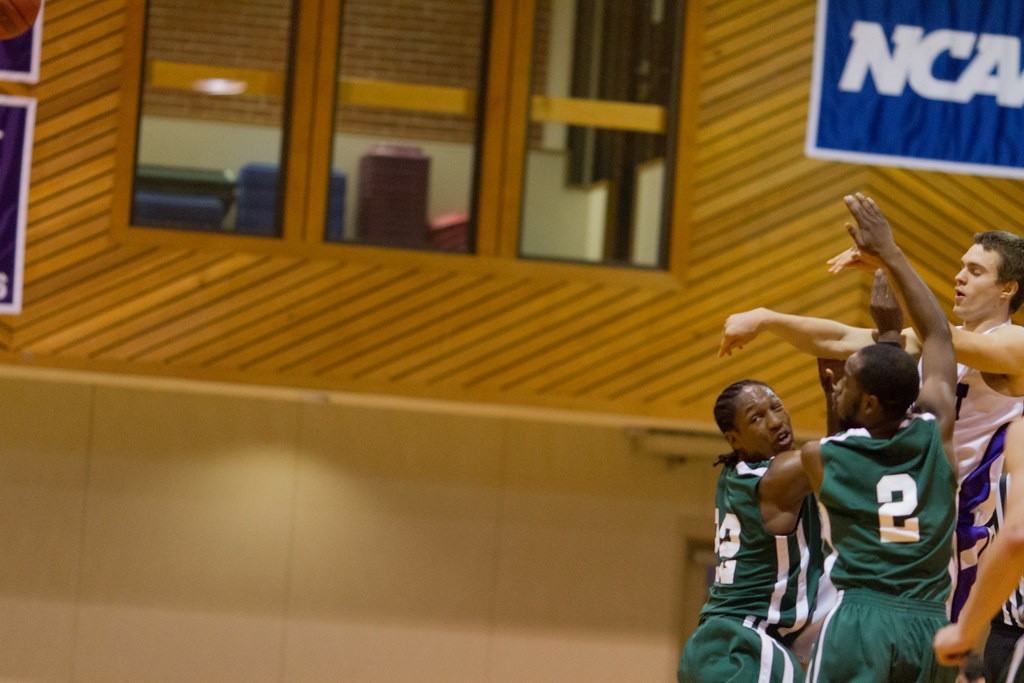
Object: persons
675 189 1024 683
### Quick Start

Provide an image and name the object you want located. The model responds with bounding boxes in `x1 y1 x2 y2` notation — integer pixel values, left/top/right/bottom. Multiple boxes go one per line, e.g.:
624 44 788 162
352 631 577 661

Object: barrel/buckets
357 143 431 250
236 161 344 241
